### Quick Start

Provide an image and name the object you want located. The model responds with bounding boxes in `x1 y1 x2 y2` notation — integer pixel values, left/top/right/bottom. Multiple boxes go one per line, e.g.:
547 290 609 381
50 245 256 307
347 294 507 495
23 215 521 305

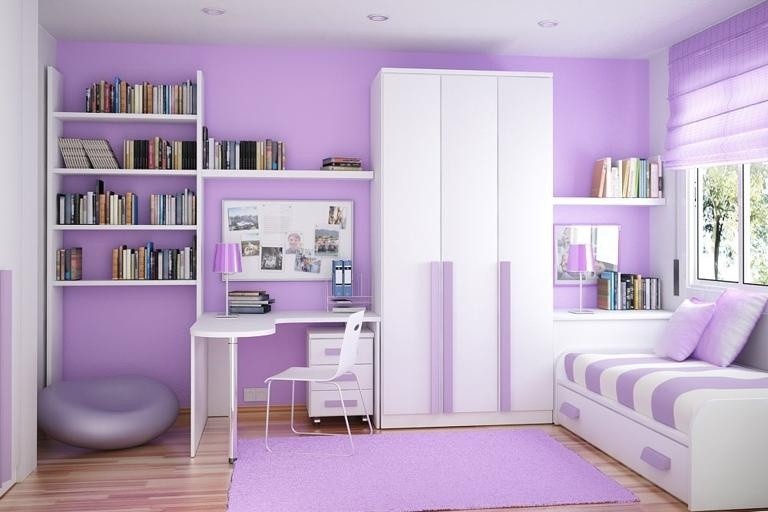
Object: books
56 76 196 280
341 260 352 296
598 269 663 310
228 290 276 314
590 156 663 199
332 260 343 295
332 299 352 304
332 304 367 313
202 122 363 170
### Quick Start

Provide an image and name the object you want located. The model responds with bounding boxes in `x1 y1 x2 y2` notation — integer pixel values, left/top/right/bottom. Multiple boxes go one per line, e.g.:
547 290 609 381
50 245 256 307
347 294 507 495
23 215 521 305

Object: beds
553 350 767 512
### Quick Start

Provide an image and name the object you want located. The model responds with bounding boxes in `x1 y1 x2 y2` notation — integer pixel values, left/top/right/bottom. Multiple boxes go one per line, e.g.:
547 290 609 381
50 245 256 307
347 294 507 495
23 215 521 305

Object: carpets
225 429 641 512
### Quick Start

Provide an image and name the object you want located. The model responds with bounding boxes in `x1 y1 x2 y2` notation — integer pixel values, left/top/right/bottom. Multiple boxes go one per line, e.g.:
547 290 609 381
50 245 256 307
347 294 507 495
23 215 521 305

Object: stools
38 377 179 451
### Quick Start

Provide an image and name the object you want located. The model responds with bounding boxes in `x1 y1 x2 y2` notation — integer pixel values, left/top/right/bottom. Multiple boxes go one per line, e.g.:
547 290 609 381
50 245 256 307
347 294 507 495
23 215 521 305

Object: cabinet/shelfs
46 65 382 458
371 67 554 430
553 196 674 321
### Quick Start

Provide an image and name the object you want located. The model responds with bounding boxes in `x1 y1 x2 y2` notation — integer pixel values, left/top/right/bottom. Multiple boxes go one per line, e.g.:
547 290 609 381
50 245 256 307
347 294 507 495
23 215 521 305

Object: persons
285 233 300 253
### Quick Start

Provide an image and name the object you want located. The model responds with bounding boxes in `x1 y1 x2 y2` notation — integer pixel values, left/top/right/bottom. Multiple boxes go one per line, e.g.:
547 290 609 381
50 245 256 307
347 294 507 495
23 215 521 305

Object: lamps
567 244 594 314
213 243 243 318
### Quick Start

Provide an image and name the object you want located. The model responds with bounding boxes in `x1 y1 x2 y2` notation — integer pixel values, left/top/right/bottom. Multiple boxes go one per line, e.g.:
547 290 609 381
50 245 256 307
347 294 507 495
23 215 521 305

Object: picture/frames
553 224 621 287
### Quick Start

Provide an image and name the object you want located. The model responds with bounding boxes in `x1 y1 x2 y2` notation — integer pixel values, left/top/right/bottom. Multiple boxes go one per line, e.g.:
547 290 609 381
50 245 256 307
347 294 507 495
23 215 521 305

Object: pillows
654 299 717 362
689 296 704 305
690 288 768 368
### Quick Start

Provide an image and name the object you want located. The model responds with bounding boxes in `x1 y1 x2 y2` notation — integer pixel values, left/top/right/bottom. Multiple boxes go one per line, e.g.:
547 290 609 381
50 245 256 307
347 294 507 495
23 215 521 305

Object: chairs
263 307 374 457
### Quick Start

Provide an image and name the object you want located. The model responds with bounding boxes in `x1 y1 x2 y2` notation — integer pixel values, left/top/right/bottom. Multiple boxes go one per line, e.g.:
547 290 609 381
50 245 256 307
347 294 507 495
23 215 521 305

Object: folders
332 260 352 296
